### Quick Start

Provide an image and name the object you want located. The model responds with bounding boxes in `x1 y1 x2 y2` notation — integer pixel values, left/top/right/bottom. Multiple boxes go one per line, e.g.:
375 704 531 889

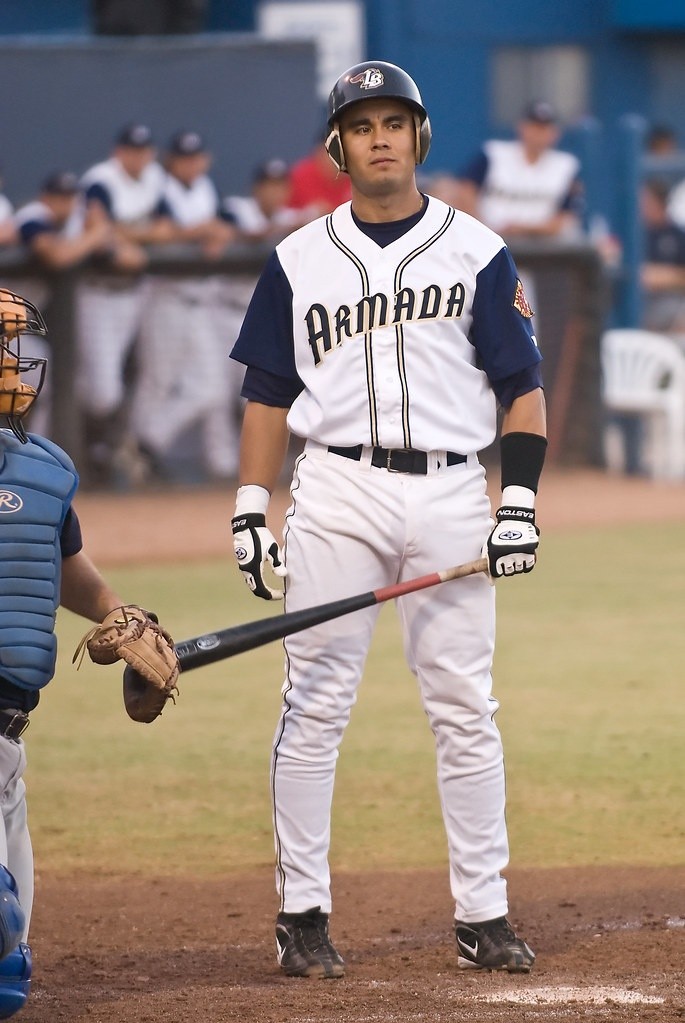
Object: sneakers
275 906 344 979
452 915 536 971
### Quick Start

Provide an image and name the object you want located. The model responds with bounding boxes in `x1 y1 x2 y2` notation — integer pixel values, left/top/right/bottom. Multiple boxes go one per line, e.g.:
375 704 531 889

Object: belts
0 710 30 741
329 442 470 474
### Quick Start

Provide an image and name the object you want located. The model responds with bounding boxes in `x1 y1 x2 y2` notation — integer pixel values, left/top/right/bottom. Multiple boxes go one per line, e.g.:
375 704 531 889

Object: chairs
596 328 684 477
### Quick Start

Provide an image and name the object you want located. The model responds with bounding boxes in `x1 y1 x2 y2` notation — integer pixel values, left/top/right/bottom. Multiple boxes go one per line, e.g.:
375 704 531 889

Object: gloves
486 483 541 578
231 485 289 601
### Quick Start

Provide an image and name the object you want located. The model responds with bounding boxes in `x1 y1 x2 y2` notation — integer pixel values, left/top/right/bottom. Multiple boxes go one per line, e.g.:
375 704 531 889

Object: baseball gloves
87 604 182 724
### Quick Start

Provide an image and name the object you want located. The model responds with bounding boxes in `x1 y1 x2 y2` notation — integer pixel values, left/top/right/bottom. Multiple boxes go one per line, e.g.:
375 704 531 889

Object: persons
0 286 180 1019
0 98 685 486
228 58 549 976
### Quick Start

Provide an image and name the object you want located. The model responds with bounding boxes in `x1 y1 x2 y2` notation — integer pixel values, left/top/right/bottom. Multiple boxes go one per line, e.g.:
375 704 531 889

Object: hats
170 132 204 156
119 125 156 147
524 100 558 125
49 171 80 192
256 156 290 184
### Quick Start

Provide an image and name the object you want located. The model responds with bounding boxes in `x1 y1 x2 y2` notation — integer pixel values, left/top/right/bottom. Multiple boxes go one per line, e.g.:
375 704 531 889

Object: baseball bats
176 558 488 673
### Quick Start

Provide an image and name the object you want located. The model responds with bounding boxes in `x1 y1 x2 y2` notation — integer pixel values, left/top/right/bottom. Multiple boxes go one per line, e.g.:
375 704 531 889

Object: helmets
322 60 436 173
